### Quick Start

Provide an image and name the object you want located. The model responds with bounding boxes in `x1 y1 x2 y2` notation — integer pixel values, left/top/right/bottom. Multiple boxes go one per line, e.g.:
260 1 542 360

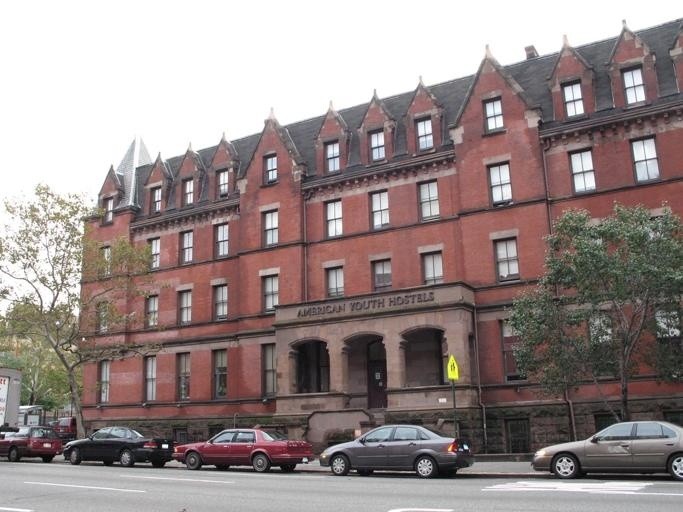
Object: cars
530 418 683 482
0 416 77 466
170 426 316 474
62 425 176 471
318 423 477 482
